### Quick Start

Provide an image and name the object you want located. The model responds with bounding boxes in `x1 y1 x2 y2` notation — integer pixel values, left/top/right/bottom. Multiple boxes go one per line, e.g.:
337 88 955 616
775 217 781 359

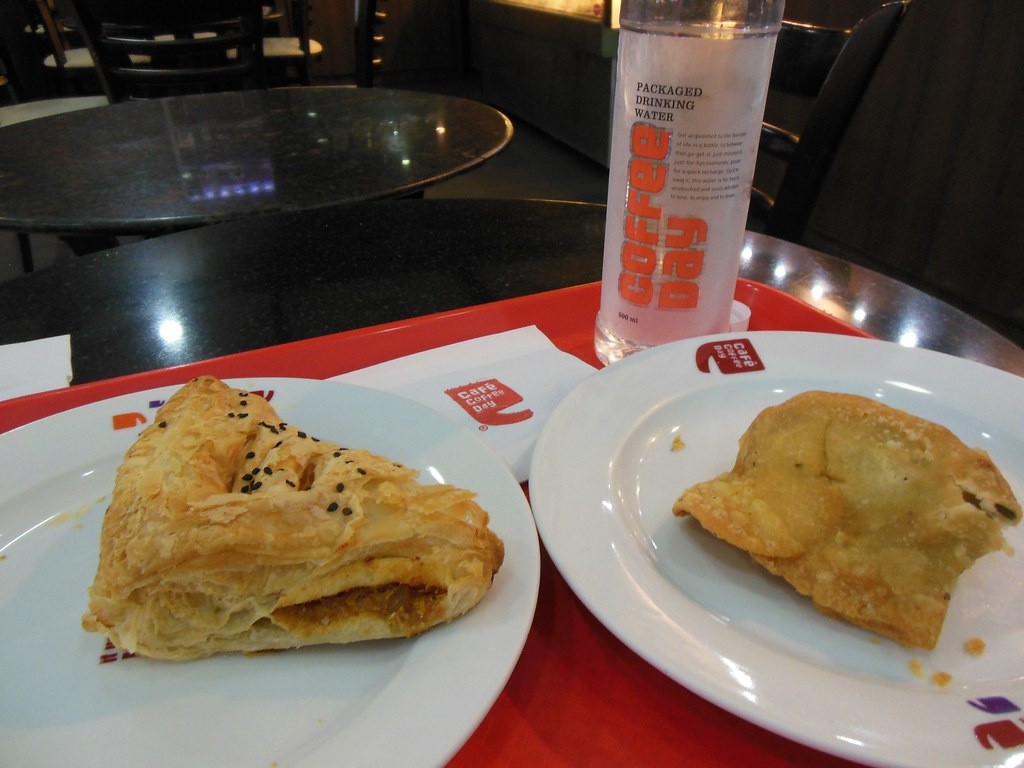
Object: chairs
67 0 313 105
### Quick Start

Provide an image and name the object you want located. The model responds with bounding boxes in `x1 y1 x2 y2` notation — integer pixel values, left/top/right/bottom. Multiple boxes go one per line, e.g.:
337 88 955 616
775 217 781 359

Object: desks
0 86 513 275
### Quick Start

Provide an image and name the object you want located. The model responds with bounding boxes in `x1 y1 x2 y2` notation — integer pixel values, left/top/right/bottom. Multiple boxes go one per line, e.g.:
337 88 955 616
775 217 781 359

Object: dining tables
0 197 1024 768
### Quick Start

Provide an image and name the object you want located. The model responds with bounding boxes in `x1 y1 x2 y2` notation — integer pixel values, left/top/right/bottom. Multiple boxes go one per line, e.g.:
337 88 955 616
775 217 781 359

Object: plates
528 330 1024 768
0 376 542 768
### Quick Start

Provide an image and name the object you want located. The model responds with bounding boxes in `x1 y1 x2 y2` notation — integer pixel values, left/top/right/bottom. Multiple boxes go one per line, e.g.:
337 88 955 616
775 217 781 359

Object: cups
595 0 787 367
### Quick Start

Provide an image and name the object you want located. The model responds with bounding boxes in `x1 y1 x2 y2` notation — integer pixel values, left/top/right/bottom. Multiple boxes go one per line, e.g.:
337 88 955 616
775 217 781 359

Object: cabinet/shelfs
746 0 911 246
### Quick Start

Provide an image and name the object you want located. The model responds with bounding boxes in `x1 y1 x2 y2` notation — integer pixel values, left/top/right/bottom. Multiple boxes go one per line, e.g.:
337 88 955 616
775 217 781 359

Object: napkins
0 334 73 402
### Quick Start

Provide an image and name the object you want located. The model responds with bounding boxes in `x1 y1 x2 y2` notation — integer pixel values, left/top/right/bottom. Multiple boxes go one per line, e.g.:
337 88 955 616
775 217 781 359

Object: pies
671 391 1023 652
87 376 506 660
